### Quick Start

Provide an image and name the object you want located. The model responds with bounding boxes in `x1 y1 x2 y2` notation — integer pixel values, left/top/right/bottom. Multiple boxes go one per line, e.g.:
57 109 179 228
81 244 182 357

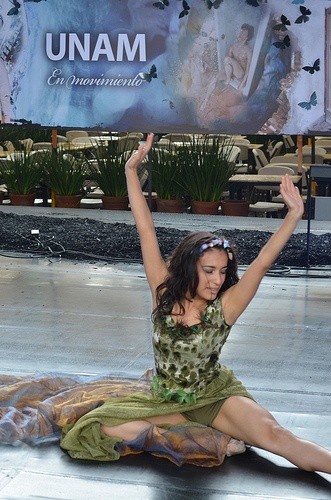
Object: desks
228 174 302 200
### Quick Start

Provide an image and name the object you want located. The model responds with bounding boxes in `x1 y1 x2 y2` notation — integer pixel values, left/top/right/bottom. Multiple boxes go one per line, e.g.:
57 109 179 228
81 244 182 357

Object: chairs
1 130 331 203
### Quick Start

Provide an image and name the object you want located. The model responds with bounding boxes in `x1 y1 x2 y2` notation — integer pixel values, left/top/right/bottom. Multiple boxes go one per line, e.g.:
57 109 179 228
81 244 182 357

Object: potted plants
0 134 251 216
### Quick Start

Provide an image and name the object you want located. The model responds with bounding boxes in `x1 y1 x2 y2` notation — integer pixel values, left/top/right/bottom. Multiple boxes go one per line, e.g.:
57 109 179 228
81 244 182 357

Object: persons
0 134 331 473
